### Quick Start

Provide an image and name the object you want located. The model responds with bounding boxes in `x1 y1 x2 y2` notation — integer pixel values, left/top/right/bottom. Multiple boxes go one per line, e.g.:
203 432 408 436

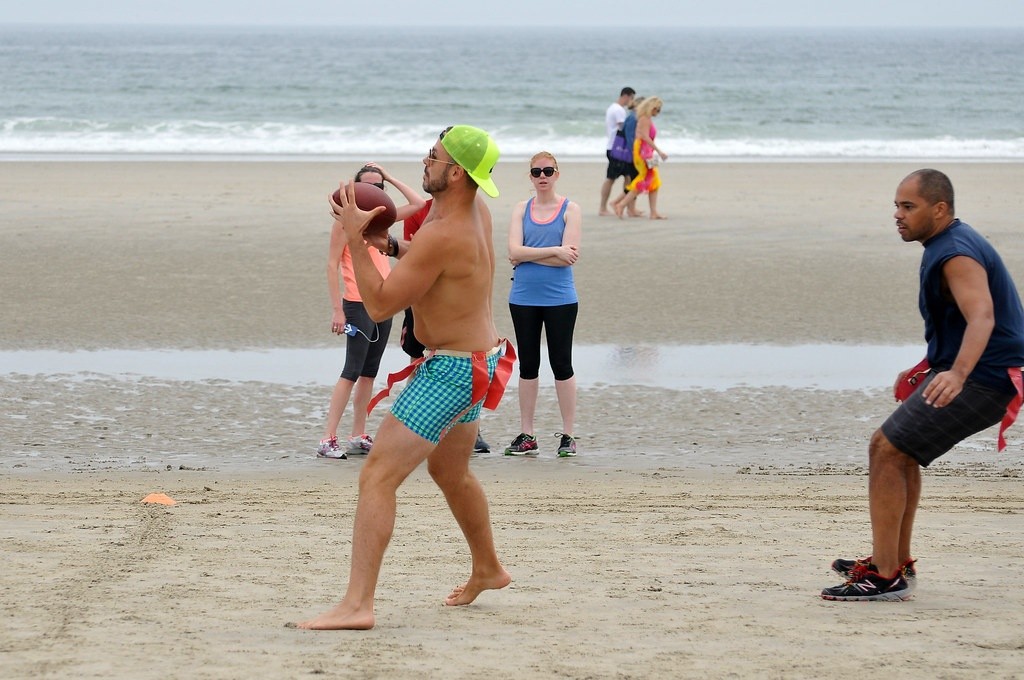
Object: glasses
656 107 661 113
373 183 384 190
531 167 558 178
428 149 460 167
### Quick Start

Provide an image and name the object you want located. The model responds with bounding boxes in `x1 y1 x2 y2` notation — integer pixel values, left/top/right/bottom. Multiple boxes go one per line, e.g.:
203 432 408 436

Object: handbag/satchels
610 127 633 164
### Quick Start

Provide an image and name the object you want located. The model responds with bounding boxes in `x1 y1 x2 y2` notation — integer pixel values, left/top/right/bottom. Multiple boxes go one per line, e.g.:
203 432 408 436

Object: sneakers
554 431 580 457
317 435 347 459
820 561 913 601
504 432 539 456
346 434 373 455
473 435 491 452
831 556 918 582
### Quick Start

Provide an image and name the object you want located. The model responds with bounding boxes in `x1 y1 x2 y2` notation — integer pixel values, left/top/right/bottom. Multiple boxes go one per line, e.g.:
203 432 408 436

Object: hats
440 125 499 198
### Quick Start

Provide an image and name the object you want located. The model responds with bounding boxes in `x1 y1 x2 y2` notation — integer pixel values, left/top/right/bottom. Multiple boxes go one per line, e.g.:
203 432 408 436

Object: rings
334 326 338 329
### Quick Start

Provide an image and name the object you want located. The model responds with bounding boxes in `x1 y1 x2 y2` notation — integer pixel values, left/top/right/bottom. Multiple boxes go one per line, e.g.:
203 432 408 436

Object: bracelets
387 235 400 257
379 233 394 257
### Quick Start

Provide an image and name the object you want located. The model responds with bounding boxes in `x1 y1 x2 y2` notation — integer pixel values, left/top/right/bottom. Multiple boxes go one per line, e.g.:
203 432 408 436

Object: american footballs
333 182 397 232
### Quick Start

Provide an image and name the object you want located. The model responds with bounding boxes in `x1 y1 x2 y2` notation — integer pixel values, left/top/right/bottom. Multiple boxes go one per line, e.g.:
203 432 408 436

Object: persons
596 85 671 221
313 161 427 460
821 168 1024 604
505 151 584 458
400 196 490 452
280 122 518 632
